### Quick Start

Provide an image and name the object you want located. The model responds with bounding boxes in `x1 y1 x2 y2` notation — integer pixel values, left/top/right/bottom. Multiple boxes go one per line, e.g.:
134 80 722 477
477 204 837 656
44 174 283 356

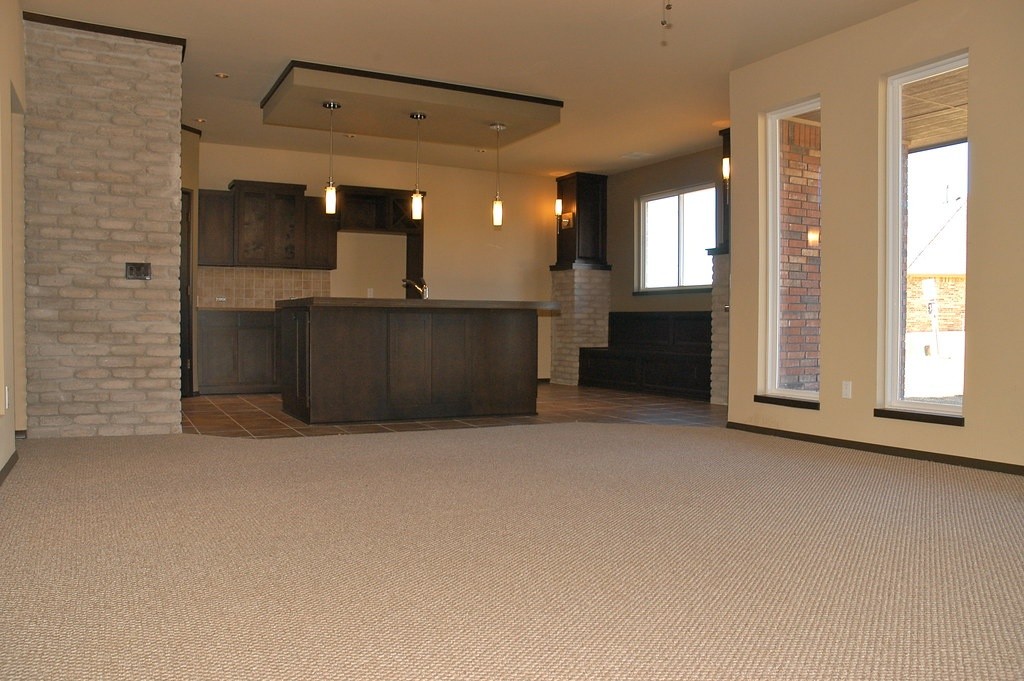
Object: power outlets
125 261 152 281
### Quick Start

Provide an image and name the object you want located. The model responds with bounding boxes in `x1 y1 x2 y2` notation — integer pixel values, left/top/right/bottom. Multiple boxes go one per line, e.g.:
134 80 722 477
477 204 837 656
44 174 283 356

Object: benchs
578 310 713 401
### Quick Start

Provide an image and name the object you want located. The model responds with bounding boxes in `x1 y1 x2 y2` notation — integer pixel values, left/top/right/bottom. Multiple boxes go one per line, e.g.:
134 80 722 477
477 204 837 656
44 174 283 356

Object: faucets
402 278 429 299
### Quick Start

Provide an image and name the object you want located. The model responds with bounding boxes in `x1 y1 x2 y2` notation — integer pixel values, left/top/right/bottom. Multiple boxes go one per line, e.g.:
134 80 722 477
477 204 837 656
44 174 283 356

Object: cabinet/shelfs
197 179 339 270
198 309 281 395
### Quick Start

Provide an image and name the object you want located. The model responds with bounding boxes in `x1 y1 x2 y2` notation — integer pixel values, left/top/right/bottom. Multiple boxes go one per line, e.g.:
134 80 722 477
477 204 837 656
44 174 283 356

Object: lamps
322 101 341 217
721 158 731 207
489 122 507 226
554 197 573 234
410 112 427 220
